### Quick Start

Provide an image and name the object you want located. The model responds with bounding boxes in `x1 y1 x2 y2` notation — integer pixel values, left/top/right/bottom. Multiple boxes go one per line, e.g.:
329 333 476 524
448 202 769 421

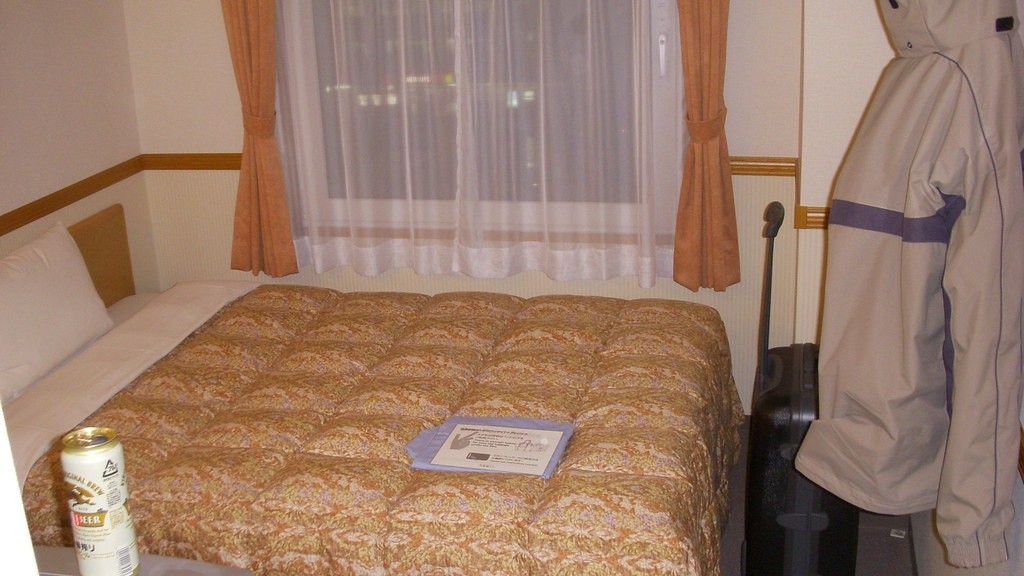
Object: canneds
61 426 141 576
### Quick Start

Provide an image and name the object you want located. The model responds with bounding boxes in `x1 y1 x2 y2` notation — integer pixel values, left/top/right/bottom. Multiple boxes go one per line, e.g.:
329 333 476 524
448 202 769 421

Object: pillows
1 219 115 409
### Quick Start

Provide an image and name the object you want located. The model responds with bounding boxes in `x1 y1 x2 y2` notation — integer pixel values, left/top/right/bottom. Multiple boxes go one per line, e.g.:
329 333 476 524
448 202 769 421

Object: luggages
738 201 859 576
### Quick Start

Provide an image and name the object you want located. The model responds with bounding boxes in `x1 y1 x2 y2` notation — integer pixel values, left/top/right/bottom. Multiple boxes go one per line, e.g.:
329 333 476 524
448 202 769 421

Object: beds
0 202 744 576
